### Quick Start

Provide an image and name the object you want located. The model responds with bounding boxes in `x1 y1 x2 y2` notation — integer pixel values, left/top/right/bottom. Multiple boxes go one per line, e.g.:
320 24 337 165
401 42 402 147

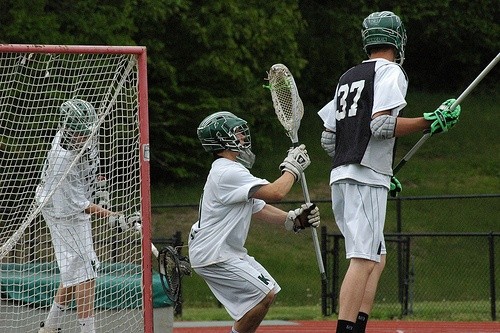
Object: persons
35 99 141 333
188 111 321 333
317 11 461 333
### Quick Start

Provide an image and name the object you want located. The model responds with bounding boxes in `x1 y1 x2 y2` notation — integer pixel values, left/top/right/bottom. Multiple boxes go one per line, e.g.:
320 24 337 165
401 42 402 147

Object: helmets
361 10 406 50
197 112 251 158
60 99 97 137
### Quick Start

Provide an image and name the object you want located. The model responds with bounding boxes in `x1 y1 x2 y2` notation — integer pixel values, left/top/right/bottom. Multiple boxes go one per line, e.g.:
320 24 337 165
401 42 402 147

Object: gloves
423 99 461 137
279 144 311 183
95 180 110 210
109 211 141 231
285 203 320 234
389 176 402 198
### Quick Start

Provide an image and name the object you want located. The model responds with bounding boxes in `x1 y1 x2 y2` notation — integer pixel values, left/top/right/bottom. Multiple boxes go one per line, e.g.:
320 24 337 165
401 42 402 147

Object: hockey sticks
267 64 327 281
392 51 500 172
132 221 183 303
